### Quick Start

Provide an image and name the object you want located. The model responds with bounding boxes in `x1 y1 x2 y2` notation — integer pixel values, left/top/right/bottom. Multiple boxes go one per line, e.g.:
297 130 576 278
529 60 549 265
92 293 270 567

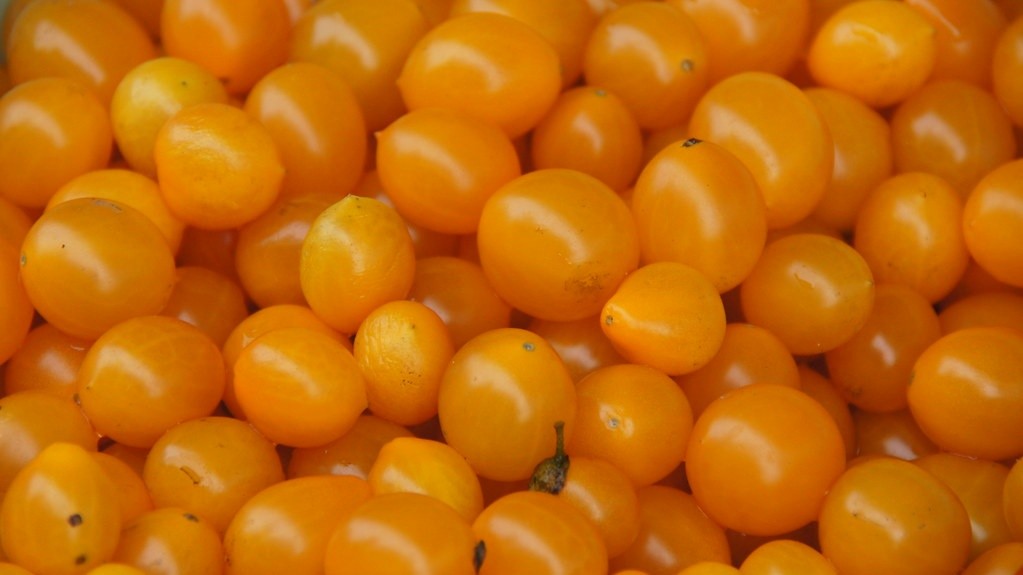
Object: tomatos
0 0 1023 575
